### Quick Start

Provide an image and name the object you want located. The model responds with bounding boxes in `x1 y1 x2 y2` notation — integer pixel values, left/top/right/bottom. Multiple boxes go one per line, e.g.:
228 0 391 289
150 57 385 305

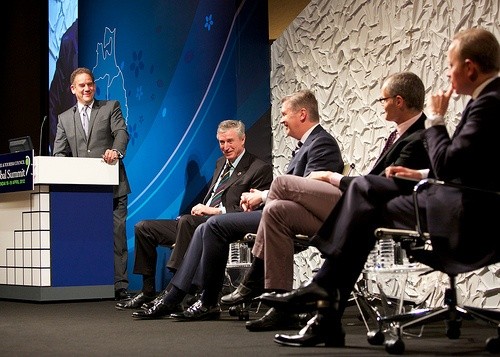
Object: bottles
367 241 379 268
379 236 394 269
229 242 240 263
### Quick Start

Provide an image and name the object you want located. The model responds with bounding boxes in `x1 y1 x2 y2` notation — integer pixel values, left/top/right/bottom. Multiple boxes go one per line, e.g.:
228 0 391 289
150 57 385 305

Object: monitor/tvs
8 136 33 153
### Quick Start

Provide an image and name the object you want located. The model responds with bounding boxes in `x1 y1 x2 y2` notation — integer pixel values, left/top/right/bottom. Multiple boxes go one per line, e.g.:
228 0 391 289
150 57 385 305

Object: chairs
366 179 500 357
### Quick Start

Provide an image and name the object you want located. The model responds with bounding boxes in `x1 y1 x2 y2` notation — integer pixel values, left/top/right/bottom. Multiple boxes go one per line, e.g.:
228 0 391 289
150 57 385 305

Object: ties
378 128 398 160
291 141 303 158
81 105 91 139
201 163 232 223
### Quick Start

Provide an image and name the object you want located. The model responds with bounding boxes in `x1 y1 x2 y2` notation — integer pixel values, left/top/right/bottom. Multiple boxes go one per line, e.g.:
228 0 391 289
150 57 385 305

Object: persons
260 28 500 347
131 90 345 321
220 72 430 333
115 120 274 312
53 67 135 301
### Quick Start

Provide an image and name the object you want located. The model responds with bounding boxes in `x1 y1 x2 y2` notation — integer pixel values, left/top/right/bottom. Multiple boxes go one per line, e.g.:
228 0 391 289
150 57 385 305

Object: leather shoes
273 315 346 348
168 300 222 321
131 291 182 320
245 307 300 331
219 283 259 306
258 282 331 310
115 288 131 301
115 291 156 311
140 290 169 310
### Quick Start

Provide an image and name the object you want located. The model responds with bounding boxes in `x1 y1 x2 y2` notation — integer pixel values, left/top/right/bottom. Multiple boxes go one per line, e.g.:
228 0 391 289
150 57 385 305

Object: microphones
39 116 47 156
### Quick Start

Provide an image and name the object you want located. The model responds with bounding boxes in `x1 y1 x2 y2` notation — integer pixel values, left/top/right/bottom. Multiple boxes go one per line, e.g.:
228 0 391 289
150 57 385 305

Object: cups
394 243 401 264
240 243 248 263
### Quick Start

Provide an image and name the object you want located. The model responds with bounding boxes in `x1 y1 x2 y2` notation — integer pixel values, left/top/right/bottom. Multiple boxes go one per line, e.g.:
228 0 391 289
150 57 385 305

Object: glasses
379 95 398 104
